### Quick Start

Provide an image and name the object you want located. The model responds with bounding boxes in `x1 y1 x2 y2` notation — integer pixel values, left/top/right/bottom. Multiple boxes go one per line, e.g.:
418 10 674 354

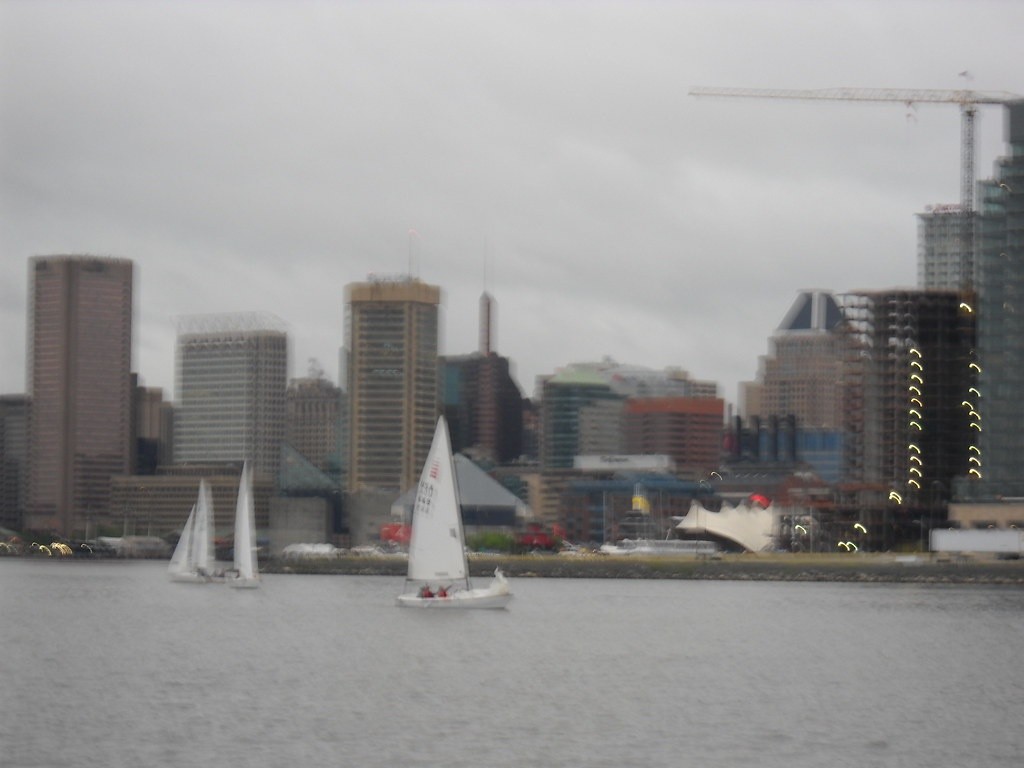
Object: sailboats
223 454 263 591
163 476 226 586
395 412 515 612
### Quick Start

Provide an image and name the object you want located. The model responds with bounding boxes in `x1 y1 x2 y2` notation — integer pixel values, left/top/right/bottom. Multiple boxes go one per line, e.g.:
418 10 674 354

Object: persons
417 584 452 598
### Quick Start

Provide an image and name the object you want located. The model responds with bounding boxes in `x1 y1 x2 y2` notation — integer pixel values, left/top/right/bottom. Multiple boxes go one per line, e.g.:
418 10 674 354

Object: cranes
691 70 1024 320
